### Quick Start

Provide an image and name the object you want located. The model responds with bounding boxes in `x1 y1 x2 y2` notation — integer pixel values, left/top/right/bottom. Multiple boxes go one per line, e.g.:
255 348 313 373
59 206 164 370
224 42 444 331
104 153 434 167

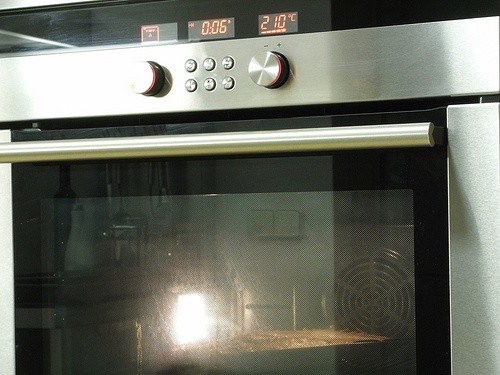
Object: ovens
0 0 500 375
